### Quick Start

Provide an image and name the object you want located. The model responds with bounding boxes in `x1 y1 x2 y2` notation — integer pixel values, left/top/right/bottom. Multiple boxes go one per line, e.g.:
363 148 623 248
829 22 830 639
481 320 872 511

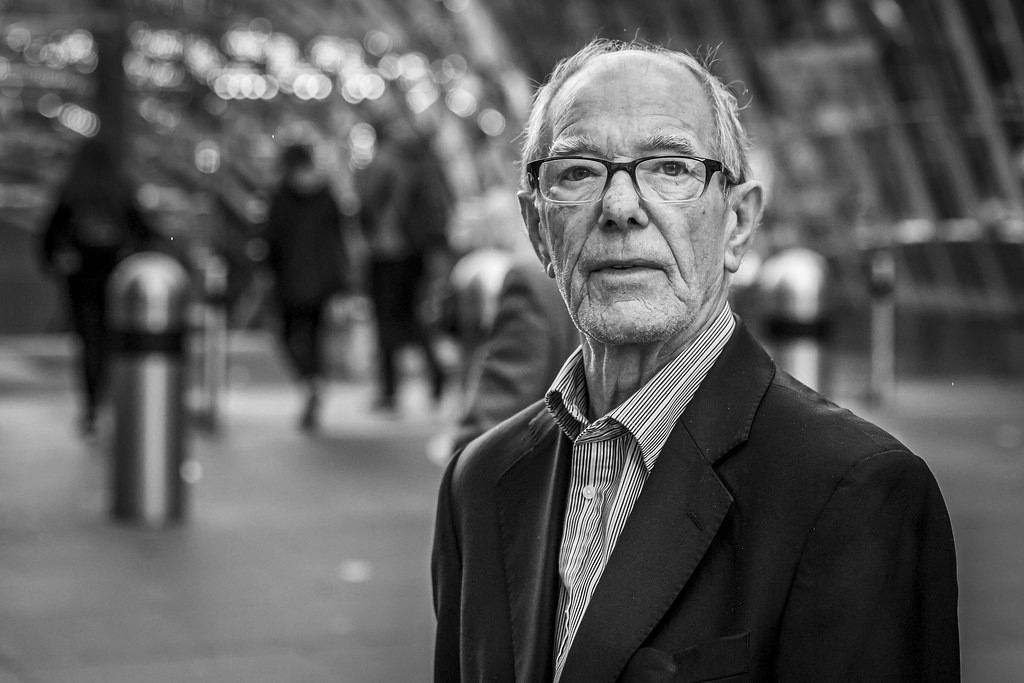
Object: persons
262 142 354 434
37 135 177 435
355 114 455 414
430 27 962 683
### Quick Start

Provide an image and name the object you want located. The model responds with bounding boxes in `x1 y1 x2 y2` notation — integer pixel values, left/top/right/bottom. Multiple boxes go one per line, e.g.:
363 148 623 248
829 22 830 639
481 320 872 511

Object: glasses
527 155 742 204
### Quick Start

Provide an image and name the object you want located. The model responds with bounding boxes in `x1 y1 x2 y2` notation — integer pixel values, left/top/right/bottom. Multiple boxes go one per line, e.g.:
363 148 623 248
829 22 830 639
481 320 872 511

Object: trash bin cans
116 261 194 528
760 246 832 390
